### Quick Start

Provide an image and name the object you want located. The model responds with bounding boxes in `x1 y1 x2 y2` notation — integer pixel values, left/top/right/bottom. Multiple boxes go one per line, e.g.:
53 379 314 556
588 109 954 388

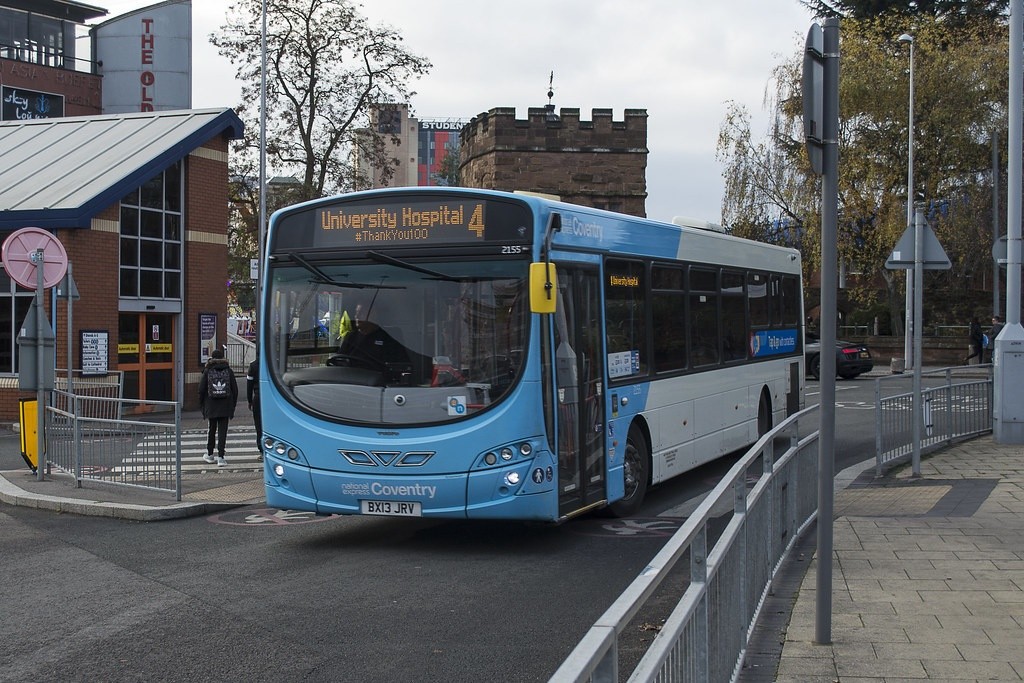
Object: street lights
897 31 915 369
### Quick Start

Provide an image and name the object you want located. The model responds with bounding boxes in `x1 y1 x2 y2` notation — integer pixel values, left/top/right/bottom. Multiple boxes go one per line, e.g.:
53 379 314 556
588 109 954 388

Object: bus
255 186 806 524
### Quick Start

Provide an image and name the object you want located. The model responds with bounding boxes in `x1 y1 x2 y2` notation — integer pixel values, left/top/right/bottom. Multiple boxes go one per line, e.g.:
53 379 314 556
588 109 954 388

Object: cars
804 340 875 379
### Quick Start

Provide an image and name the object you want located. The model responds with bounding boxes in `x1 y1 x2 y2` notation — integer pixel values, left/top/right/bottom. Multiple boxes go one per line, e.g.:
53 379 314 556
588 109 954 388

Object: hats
212 350 222 359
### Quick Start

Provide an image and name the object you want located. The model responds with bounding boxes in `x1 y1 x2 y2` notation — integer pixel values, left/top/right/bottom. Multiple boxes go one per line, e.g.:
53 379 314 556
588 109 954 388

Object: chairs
479 310 746 400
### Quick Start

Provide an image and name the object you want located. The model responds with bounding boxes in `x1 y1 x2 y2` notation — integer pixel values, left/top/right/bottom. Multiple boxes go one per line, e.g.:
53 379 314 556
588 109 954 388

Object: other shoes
257 454 263 462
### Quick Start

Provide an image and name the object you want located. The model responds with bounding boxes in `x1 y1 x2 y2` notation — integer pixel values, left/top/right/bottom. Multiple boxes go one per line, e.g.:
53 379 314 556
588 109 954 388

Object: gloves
248 401 253 411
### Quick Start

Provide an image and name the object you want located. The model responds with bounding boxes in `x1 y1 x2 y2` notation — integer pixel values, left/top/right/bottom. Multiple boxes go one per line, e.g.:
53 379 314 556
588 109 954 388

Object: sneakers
218 457 228 466
203 454 214 463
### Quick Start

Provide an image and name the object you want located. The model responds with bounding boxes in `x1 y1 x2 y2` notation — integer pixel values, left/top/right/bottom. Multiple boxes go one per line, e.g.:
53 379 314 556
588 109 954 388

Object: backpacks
208 367 231 398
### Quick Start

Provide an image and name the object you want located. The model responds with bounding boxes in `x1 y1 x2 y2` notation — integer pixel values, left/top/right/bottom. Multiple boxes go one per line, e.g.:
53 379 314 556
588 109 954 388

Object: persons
964 317 983 368
988 316 1004 350
247 359 266 464
199 349 238 466
337 299 413 379
